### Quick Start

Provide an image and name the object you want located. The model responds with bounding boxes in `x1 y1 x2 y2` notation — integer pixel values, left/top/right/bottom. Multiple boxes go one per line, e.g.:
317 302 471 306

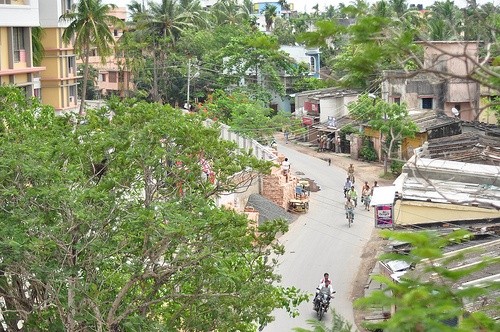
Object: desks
289 197 309 213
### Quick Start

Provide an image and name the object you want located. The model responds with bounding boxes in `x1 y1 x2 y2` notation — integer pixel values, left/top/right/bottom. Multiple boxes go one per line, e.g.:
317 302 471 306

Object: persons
282 158 291 184
316 135 340 152
284 127 291 144
344 178 353 198
348 186 358 207
345 196 354 223
362 181 379 211
313 273 334 313
348 163 355 184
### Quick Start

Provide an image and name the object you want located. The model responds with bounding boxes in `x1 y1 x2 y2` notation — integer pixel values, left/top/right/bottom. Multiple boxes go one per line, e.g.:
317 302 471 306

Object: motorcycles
312 287 336 321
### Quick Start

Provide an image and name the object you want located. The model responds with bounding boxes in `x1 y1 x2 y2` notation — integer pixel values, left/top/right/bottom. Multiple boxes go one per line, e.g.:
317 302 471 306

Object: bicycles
344 173 371 228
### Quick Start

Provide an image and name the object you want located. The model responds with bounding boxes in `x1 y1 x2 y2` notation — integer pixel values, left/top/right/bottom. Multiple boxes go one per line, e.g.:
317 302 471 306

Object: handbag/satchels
361 196 363 202
